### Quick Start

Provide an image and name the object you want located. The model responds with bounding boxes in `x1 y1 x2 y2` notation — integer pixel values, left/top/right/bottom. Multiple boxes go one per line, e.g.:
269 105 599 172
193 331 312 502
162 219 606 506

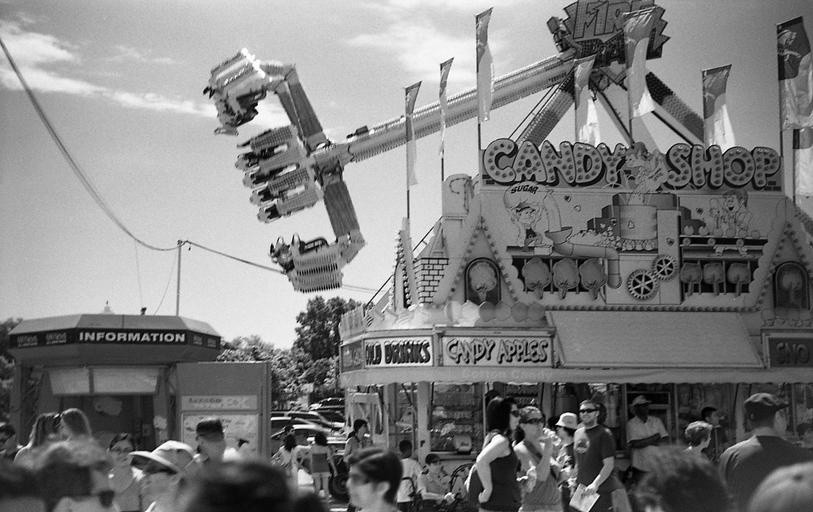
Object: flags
623 5 654 119
701 64 735 151
403 81 419 189
777 15 813 131
473 8 494 121
792 125 812 199
573 53 602 144
435 56 452 156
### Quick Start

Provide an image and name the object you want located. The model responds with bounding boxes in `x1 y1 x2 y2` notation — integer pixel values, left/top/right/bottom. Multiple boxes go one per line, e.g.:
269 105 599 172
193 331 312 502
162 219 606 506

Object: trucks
303 436 351 500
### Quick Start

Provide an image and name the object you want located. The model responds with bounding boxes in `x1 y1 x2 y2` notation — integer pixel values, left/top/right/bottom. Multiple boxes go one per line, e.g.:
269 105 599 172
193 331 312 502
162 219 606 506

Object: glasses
347 473 371 484
580 409 598 413
526 417 545 424
510 410 519 418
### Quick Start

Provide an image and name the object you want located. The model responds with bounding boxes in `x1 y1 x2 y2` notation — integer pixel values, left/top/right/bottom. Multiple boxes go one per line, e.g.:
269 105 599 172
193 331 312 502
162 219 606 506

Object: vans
270 411 344 432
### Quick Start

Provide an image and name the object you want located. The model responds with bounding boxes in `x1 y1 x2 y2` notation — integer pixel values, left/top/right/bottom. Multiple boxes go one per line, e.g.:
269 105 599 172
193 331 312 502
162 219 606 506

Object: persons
792 422 812 450
547 414 559 431
1 409 449 511
625 395 670 481
701 406 719 458
513 405 563 512
632 456 740 512
746 460 812 511
681 419 714 460
474 395 520 512
571 398 616 512
714 392 796 503
554 411 579 468
201 85 304 268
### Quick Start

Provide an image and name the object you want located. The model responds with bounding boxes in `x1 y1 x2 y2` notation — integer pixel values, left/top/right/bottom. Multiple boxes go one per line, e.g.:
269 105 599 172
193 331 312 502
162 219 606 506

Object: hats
555 413 578 429
629 395 651 417
744 393 790 420
129 440 201 482
196 417 228 435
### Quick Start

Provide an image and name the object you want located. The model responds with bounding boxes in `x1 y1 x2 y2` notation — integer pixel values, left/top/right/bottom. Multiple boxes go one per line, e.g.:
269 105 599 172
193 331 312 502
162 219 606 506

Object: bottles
541 428 560 446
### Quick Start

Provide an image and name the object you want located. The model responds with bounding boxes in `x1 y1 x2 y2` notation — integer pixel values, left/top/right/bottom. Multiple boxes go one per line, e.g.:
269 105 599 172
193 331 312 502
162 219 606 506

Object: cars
311 405 344 412
307 397 344 410
270 425 330 458
270 417 317 440
318 410 344 423
